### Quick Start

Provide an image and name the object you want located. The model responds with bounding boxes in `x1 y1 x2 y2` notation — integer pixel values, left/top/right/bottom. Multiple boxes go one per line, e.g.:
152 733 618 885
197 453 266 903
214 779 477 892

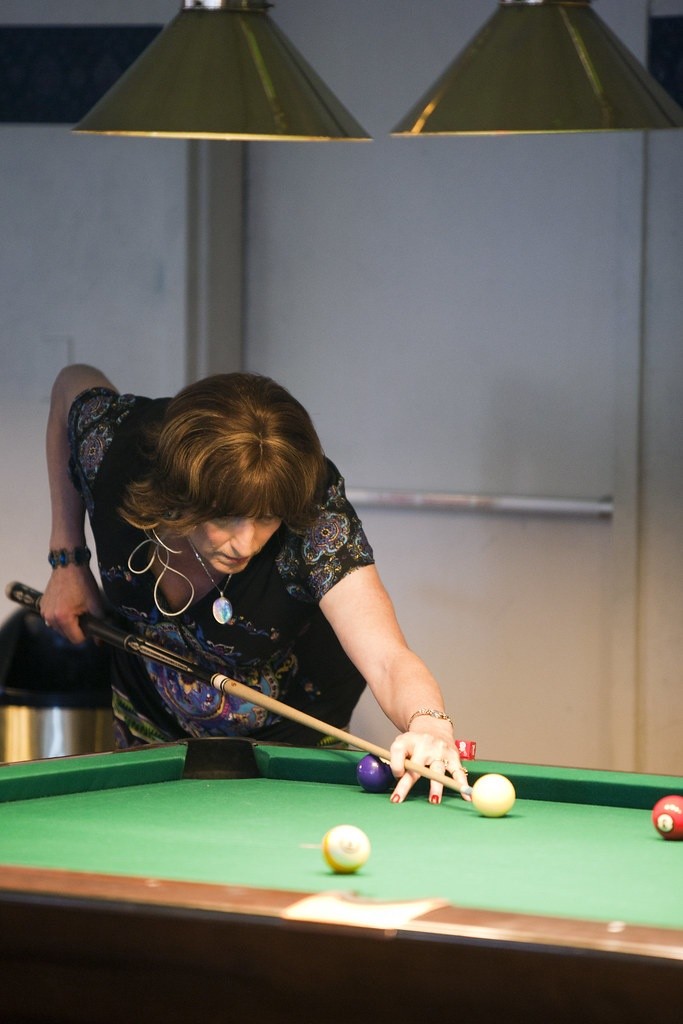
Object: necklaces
186 536 233 624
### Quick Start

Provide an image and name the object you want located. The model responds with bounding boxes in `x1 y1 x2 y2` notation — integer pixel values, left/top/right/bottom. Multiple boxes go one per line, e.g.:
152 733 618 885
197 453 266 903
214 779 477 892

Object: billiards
356 752 400 795
652 794 683 841
474 772 516 818
322 824 374 872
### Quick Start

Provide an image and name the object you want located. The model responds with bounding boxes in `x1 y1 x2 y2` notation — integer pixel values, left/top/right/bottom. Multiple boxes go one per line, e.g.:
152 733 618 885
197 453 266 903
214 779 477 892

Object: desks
0 734 683 1024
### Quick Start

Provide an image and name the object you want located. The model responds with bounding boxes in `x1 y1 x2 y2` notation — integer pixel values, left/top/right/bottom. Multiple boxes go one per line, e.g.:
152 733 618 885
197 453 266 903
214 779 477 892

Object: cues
5 577 474 799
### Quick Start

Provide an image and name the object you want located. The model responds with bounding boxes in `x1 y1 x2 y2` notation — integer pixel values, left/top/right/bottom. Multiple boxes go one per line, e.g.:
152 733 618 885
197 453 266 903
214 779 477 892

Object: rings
451 767 468 777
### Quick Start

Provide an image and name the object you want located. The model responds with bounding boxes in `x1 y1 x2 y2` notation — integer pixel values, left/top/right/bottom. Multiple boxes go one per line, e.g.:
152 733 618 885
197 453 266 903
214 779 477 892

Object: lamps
72 0 373 142
387 0 683 136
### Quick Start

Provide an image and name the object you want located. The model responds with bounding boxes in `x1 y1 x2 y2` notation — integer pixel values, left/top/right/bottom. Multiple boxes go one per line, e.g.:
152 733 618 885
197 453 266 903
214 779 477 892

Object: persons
40 363 474 804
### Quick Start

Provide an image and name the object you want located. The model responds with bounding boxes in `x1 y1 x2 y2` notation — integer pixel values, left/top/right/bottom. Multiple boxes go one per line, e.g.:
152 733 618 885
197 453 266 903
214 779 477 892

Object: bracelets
48 544 91 570
407 708 453 731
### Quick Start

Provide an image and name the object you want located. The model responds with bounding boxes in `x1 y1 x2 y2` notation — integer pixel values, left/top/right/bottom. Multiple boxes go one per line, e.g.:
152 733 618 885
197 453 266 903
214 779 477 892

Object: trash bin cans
0 604 118 765
